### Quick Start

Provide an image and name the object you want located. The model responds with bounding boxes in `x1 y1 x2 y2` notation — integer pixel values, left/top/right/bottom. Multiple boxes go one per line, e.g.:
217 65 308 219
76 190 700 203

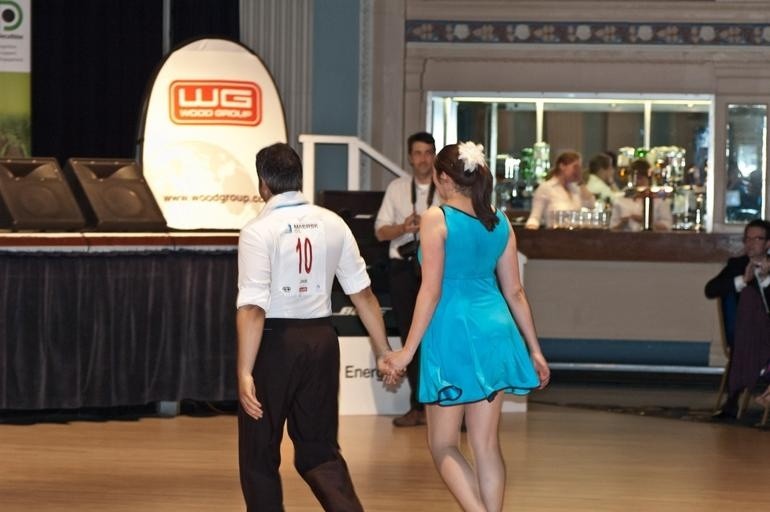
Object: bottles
617 147 706 232
497 141 550 209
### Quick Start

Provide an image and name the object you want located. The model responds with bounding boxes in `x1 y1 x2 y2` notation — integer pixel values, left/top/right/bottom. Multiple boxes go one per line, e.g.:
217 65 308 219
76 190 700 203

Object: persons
381 141 551 512
236 142 406 512
524 150 595 230
583 152 619 203
609 160 672 231
373 133 467 433
704 219 770 419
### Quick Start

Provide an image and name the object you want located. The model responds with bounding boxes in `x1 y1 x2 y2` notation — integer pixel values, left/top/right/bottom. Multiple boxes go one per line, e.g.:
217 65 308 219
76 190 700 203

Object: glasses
742 236 767 243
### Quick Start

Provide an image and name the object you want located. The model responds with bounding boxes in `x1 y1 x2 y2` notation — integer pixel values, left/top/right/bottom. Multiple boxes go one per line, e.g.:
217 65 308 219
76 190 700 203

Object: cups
544 211 609 230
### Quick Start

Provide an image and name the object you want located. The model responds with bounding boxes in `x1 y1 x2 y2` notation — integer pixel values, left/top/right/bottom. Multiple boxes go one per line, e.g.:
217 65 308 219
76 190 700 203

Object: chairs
714 297 769 421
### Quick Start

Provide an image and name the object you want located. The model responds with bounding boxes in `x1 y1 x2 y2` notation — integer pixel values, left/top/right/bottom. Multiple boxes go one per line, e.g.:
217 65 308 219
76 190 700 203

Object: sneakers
393 408 427 426
